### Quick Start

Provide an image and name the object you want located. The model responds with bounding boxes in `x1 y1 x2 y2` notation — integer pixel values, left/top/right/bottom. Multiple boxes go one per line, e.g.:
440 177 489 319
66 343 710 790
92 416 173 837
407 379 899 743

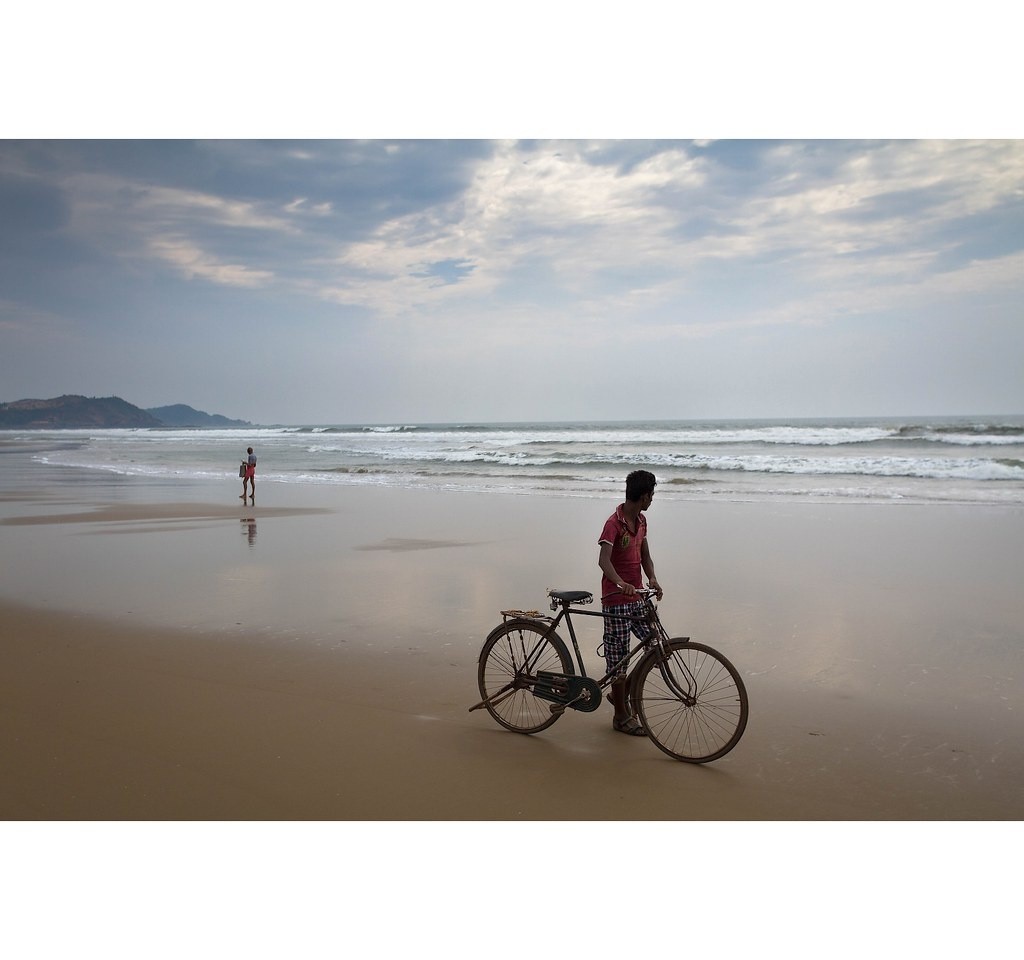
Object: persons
597 470 664 736
239 447 257 499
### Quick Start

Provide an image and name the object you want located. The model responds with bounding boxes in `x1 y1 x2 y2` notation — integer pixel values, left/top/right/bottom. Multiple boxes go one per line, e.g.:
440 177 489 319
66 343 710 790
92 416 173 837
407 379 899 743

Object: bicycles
468 582 750 764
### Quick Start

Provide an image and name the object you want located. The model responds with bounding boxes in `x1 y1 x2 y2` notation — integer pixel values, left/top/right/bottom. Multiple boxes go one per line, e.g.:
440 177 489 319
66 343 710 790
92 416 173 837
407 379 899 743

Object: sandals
607 692 632 715
613 714 647 736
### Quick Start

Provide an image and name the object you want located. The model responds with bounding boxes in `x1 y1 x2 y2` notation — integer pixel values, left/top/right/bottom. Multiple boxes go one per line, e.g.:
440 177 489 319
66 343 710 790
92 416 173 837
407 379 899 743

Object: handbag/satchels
238 462 247 477
